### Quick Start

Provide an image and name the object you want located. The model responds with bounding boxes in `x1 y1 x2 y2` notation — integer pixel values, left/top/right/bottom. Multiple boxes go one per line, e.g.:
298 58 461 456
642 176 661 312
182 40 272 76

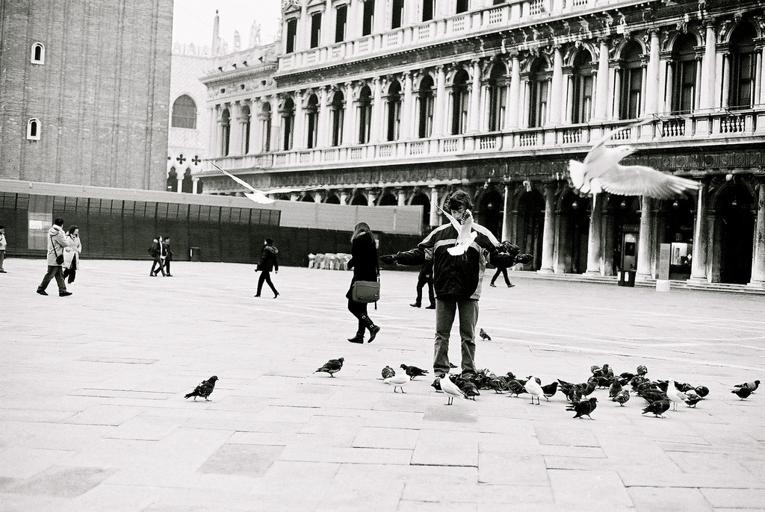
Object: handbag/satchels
56 254 64 265
353 279 380 302
147 247 157 257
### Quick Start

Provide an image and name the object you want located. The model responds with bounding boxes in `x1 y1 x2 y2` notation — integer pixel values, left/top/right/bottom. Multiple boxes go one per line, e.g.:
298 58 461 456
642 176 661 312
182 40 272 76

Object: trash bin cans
190 247 201 261
617 269 636 287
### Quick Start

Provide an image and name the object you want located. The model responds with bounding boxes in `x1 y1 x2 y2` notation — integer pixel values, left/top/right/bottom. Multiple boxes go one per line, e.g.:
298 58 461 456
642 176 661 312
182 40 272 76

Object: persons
154 236 173 277
0 226 7 273
37 218 73 296
344 222 380 344
254 239 279 299
62 225 82 285
379 190 510 385
409 229 436 309
490 241 516 287
148 236 170 277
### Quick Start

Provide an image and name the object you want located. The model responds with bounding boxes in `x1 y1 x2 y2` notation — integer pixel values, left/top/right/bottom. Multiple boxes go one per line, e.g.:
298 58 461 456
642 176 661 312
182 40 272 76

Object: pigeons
313 357 344 378
184 375 219 403
479 328 491 341
568 126 702 200
382 363 559 405
211 161 320 206
435 204 478 259
557 362 709 420
730 379 761 402
